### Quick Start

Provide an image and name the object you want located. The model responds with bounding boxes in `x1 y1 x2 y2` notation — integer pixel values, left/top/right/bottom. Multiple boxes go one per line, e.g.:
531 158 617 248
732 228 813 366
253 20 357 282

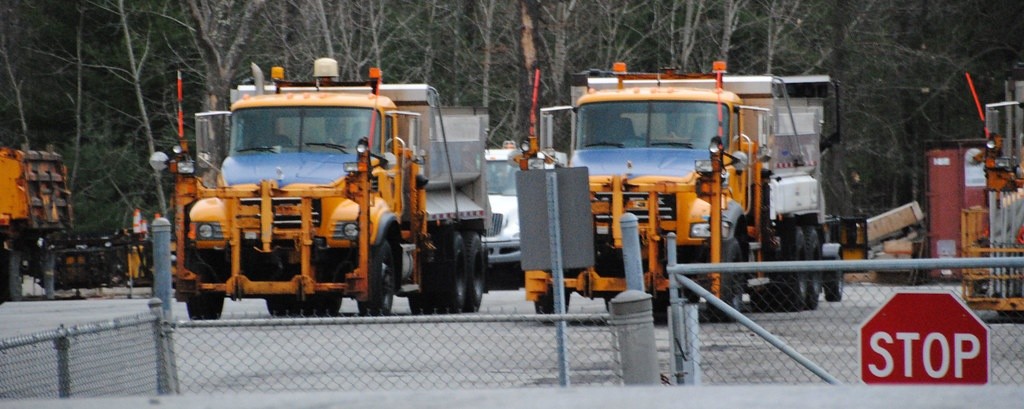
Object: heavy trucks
143 55 494 321
525 62 870 321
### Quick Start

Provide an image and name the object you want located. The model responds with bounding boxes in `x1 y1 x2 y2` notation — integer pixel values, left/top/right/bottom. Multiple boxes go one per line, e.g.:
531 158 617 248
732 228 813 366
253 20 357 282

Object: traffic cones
132 208 160 241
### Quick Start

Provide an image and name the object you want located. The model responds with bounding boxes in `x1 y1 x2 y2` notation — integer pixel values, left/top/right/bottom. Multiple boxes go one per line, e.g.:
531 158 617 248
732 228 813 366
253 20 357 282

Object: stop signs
857 289 990 387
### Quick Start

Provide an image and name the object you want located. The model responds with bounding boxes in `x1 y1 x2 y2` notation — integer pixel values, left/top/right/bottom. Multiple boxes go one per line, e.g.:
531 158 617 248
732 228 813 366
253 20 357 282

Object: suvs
479 138 533 290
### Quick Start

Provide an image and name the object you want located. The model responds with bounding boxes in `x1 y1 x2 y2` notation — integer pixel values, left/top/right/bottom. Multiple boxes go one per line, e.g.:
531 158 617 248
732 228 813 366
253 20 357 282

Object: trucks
955 65 1024 324
0 145 76 304
